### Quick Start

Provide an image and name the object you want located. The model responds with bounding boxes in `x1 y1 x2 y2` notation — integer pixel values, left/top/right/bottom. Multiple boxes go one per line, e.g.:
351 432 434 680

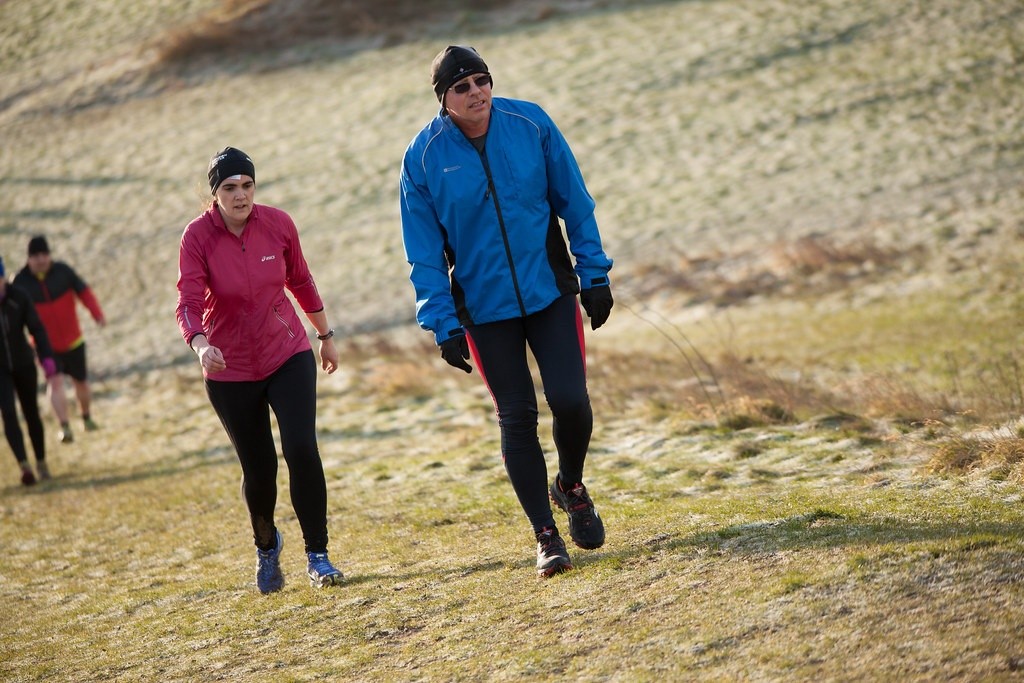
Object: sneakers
61 431 73 443
84 421 98 432
21 470 36 486
306 551 345 587
255 530 284 597
37 466 50 480
536 528 574 579
546 473 606 550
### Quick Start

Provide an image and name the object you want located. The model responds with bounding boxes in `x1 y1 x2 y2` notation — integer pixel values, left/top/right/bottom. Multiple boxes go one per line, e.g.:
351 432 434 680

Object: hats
207 146 256 195
28 236 50 257
0 258 5 278
429 44 490 102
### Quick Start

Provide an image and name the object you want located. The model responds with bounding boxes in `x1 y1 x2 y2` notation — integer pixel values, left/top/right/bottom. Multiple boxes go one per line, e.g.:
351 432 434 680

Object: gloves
580 283 614 331
438 334 473 373
39 352 58 381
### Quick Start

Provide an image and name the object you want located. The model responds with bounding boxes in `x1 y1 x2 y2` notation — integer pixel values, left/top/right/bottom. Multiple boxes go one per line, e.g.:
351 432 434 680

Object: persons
399 45 615 578
173 147 344 595
10 236 107 446
0 257 50 486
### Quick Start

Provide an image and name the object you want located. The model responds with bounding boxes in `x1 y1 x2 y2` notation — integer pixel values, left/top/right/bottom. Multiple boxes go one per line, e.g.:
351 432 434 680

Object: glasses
447 73 492 94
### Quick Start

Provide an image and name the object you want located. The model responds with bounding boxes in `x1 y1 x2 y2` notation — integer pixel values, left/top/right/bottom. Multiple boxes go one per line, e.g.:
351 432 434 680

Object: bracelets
316 329 334 340
